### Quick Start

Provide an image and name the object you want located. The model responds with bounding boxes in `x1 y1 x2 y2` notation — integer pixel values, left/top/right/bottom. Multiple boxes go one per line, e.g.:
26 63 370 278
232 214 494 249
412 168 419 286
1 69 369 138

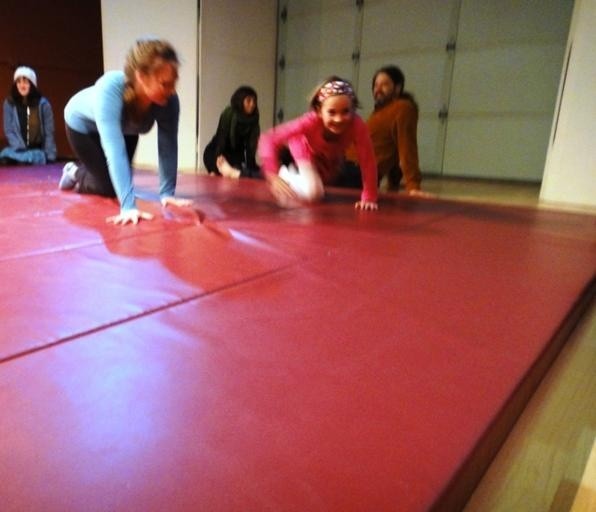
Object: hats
14 66 37 88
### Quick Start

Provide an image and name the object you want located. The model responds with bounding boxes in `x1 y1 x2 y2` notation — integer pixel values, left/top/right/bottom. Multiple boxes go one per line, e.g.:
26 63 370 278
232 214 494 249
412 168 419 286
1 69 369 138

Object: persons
254 75 379 212
216 63 440 198
202 85 262 176
1 67 58 168
59 39 196 226
